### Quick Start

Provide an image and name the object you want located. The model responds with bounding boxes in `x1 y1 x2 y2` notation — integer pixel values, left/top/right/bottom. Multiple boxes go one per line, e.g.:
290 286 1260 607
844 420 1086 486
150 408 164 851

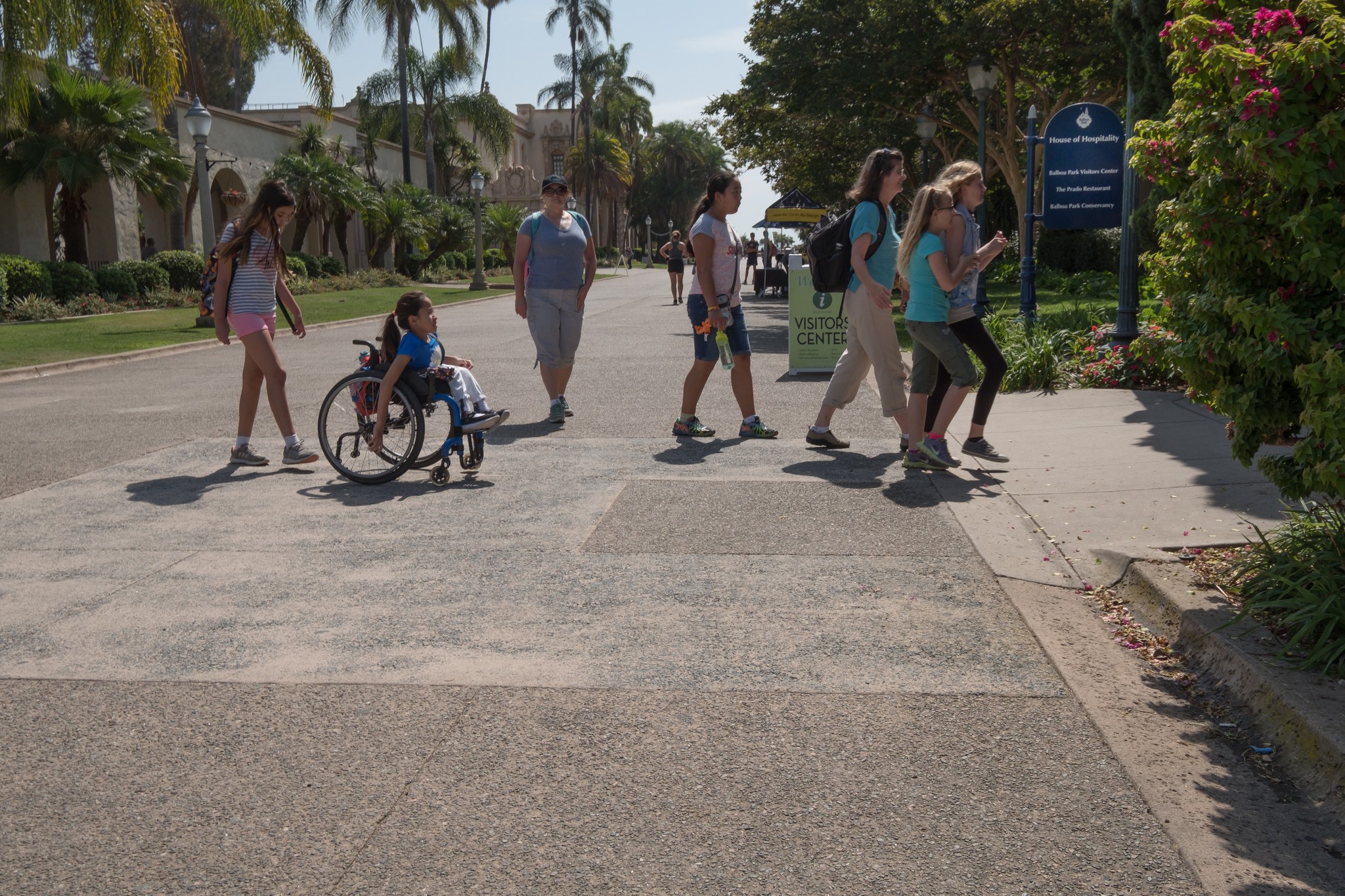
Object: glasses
542 175 568 190
934 204 954 211
880 147 892 169
883 169 904 176
543 186 568 197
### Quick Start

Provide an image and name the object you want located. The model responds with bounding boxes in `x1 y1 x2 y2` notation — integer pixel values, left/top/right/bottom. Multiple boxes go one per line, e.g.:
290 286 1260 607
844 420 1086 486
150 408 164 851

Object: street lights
915 102 936 185
469 167 489 291
966 52 999 318
184 92 217 329
668 219 674 255
645 214 654 269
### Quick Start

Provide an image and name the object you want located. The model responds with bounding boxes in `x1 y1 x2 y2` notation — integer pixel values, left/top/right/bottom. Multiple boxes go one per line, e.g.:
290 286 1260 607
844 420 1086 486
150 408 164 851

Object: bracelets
707 306 720 311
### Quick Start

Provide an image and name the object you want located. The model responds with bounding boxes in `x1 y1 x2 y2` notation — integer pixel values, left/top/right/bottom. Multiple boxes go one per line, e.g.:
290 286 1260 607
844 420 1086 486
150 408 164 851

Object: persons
806 149 910 452
760 248 764 258
762 231 772 268
627 247 632 269
672 172 778 437
896 185 982 470
923 159 1010 466
367 291 510 454
214 178 320 466
659 230 688 305
513 174 597 422
741 232 759 285
743 248 746 259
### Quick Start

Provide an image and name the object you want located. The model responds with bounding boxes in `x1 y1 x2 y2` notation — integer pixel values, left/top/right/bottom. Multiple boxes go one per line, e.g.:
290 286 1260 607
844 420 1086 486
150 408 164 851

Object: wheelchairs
316 336 484 485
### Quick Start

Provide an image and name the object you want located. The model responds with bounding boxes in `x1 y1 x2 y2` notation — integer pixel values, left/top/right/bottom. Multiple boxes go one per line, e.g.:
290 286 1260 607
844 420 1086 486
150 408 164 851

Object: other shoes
742 281 748 285
751 280 755 285
673 300 677 305
678 297 683 303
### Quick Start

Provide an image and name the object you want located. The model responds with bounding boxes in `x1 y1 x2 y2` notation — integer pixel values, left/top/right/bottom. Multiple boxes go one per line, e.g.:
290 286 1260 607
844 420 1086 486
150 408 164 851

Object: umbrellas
752 219 812 269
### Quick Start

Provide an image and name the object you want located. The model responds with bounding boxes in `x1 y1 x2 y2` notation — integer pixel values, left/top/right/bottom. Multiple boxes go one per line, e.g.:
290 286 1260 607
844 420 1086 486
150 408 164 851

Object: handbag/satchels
716 294 734 327
349 355 382 417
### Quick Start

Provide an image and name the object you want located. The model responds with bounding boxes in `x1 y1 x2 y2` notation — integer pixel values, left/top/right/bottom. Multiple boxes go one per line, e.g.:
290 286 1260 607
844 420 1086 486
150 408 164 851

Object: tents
763 187 827 298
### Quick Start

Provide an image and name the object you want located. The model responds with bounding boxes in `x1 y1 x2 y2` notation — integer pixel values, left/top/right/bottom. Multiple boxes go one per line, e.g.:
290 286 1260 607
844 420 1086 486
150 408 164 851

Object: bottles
715 330 735 370
357 351 370 368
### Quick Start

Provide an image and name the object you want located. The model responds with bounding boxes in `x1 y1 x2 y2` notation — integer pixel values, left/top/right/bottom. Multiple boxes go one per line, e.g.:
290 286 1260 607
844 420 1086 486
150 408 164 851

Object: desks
789 254 849 375
754 268 789 298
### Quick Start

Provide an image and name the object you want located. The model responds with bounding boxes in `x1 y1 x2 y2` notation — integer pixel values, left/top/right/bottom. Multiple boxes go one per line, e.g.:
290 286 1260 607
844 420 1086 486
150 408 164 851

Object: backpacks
198 217 244 319
768 239 778 256
806 199 886 293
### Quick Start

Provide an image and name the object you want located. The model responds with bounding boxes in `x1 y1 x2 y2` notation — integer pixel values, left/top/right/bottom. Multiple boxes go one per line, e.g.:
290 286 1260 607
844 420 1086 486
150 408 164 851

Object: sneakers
739 416 778 437
962 438 1009 463
900 434 909 452
806 425 850 448
672 416 715 437
951 456 961 465
482 409 510 433
229 443 270 465
549 400 565 423
901 449 949 470
282 438 319 465
460 411 500 432
916 433 962 468
559 396 574 416
